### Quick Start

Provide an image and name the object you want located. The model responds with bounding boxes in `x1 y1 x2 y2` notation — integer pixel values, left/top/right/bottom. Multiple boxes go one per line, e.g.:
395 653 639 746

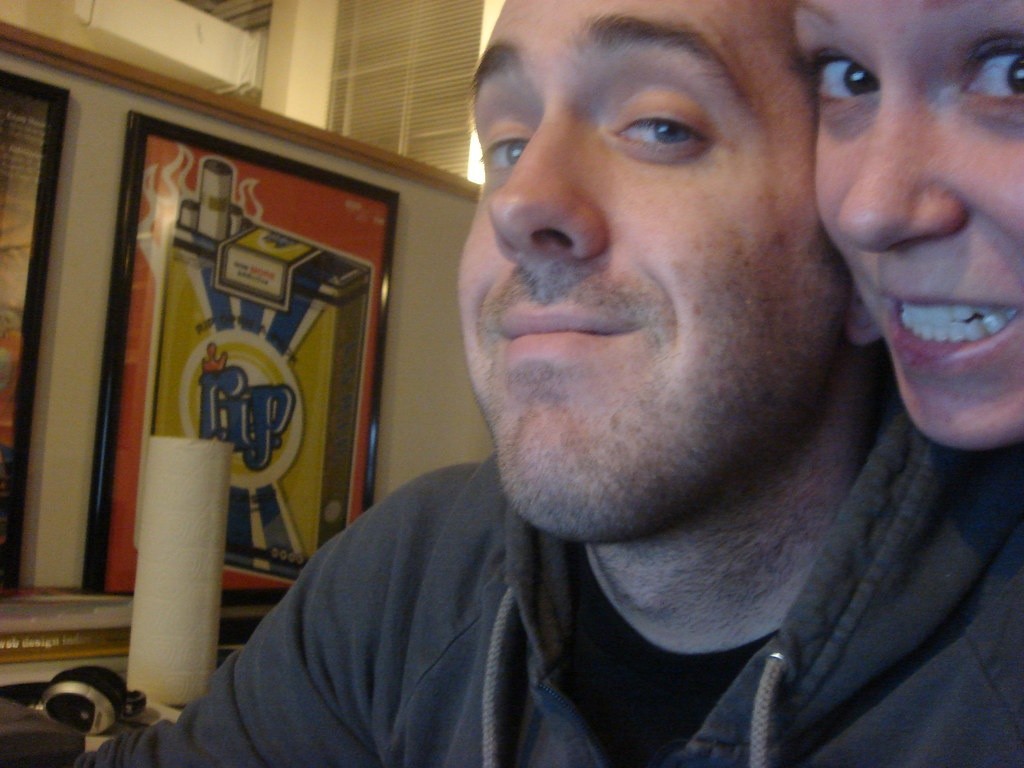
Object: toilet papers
127 436 234 705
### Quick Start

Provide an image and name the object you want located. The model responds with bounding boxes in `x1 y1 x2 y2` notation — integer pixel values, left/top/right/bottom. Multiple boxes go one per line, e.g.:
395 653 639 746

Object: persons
70 0 1024 768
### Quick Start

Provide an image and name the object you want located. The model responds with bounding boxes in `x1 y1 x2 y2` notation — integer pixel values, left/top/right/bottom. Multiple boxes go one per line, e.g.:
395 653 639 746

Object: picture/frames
0 69 73 593
78 109 404 605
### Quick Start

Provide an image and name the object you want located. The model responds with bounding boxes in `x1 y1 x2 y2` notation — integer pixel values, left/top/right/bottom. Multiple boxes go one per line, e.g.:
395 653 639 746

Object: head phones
28 664 126 736
